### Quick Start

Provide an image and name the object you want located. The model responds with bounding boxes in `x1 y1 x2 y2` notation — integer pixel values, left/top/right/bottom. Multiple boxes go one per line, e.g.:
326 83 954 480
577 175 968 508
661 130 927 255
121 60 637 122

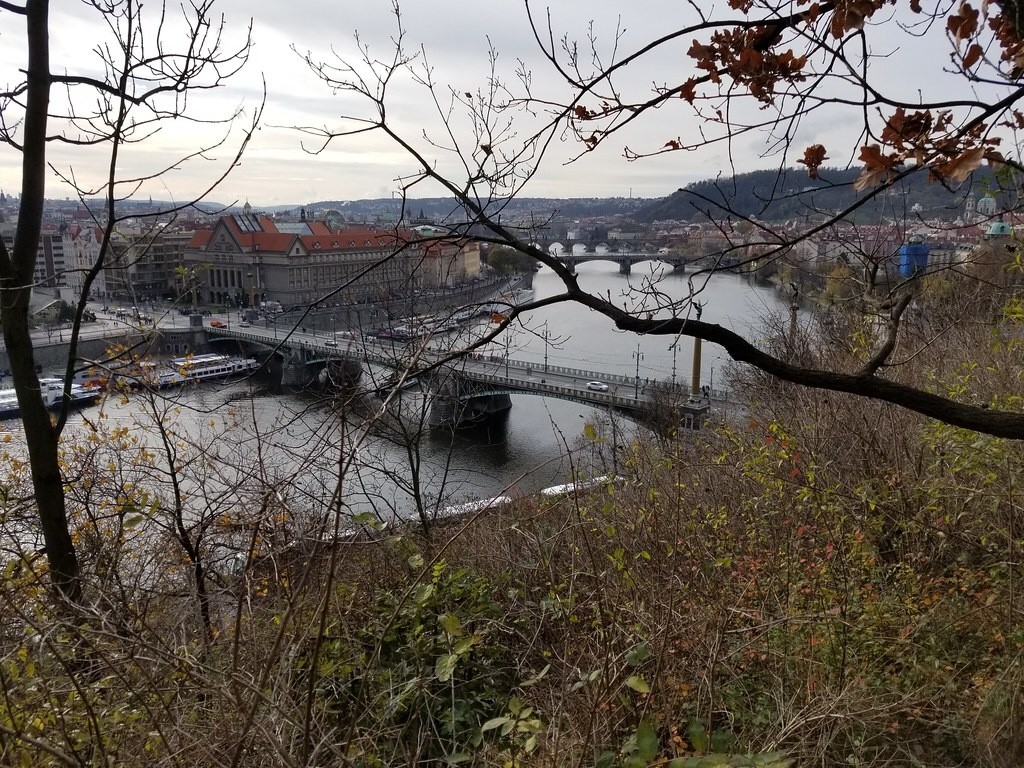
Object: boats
367 288 534 341
376 372 418 398
0 353 261 417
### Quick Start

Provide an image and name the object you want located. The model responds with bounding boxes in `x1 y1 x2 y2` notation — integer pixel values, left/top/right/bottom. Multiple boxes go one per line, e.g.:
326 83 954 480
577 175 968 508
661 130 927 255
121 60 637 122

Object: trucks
211 320 227 329
260 301 283 313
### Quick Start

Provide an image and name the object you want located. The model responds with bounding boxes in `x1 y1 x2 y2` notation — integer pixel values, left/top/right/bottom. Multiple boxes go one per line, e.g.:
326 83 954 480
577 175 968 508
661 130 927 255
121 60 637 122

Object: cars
587 381 609 392
239 322 249 327
324 340 338 347
101 305 145 320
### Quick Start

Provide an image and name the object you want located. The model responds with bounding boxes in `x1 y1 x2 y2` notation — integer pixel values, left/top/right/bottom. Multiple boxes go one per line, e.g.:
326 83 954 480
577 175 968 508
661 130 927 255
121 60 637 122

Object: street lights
331 309 337 349
667 337 681 392
711 357 721 396
503 327 512 383
632 343 644 400
225 296 231 330
237 313 240 323
542 323 551 372
